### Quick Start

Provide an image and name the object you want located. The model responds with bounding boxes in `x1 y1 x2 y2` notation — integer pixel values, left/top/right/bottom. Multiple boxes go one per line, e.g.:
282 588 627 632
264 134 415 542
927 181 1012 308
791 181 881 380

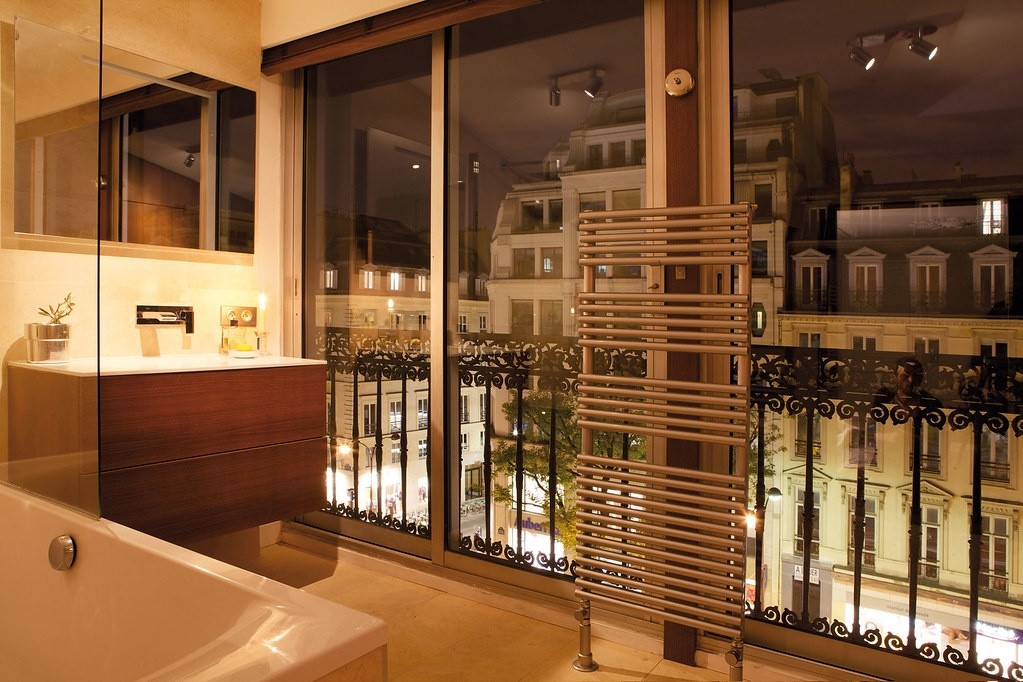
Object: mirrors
366 126 467 274
12 16 256 255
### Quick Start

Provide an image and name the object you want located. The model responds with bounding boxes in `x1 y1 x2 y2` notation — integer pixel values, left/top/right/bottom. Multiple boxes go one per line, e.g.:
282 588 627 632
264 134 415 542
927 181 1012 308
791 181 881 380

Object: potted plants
24 293 77 365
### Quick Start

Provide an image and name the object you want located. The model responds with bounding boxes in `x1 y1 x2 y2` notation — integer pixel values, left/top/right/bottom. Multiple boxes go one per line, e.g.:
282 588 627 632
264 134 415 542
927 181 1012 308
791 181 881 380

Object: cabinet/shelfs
6 363 326 546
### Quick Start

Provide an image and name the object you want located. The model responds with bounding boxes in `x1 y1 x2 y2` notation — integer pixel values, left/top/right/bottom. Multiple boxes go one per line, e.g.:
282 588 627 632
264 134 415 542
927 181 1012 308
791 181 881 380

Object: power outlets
221 305 257 326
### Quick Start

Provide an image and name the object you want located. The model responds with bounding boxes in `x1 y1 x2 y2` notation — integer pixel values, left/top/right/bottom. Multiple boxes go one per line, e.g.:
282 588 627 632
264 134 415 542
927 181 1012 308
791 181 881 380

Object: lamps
548 79 561 108
583 71 604 97
850 39 876 72
908 28 938 60
183 151 195 169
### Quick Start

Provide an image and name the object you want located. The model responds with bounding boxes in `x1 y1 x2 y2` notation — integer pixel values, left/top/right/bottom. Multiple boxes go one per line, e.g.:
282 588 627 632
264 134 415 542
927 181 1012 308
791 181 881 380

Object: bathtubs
0 480 389 682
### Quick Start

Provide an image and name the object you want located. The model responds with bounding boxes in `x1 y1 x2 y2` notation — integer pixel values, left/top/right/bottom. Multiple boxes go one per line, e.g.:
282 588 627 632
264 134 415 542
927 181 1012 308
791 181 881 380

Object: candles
257 293 265 330
